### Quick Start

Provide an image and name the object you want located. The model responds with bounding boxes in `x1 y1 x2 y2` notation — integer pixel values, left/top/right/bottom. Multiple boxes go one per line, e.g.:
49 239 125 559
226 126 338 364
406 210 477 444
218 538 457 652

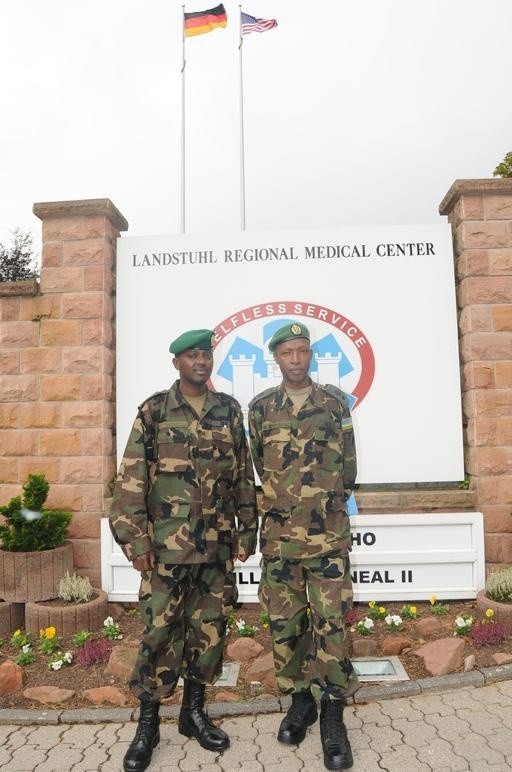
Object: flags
239 13 279 38
183 3 229 39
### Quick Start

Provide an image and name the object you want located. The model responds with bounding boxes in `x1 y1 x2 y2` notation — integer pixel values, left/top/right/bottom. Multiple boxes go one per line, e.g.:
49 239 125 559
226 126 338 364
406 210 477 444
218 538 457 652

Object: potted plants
476 565 512 635
1 471 110 641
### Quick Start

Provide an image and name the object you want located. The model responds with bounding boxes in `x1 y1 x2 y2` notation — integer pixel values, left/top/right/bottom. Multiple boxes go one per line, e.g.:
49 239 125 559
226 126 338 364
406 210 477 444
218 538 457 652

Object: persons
107 326 258 771
248 322 360 772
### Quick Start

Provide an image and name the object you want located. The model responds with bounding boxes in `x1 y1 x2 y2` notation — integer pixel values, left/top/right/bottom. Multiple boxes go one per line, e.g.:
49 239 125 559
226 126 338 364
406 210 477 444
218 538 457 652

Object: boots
123 700 161 772
320 695 354 771
178 678 230 751
276 686 318 747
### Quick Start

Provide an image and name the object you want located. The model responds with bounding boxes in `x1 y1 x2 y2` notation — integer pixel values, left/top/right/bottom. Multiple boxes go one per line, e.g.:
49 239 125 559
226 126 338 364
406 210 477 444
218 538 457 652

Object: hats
268 322 312 353
169 328 217 354
